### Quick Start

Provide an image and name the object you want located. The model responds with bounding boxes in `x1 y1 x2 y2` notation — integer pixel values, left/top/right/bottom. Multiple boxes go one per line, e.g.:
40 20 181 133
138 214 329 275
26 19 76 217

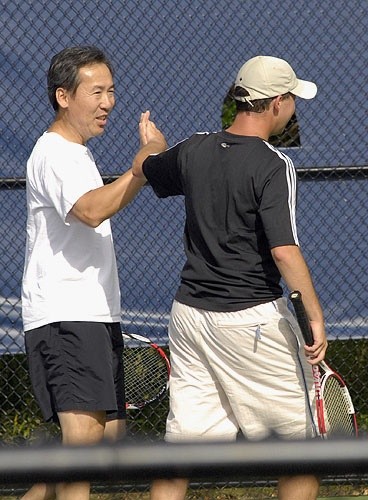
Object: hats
232 55 318 108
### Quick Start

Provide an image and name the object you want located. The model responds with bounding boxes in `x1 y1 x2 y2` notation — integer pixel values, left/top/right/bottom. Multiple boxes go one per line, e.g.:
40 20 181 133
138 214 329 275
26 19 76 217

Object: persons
18 44 150 499
132 54 329 499
